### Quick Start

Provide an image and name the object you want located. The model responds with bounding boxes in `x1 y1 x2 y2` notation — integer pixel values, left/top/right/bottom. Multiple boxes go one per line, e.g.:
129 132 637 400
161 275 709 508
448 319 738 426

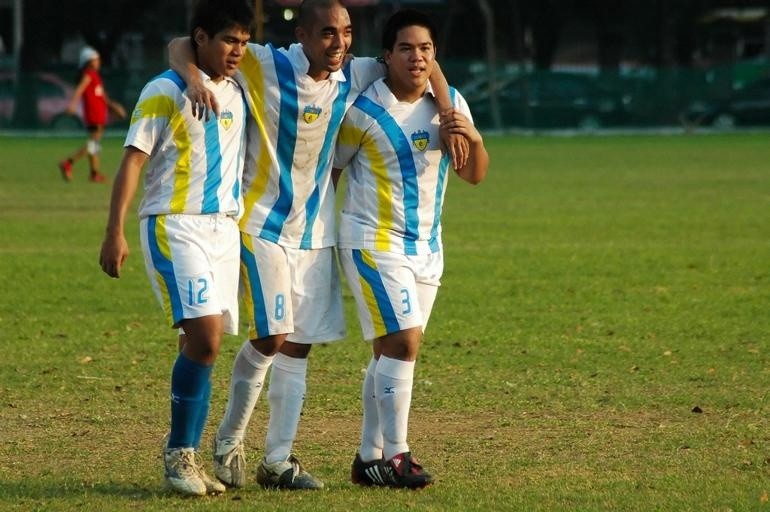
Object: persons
331 9 491 489
99 1 257 498
168 1 468 489
60 45 128 182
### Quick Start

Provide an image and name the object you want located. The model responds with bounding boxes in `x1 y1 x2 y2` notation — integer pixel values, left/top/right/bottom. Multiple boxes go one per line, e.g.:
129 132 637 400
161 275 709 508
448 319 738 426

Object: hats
79 48 98 68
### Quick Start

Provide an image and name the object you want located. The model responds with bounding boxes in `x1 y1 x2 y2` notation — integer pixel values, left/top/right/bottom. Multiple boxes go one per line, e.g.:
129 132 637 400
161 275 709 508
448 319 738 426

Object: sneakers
352 450 435 490
165 436 245 495
89 174 106 182
256 454 323 490
60 159 72 182
